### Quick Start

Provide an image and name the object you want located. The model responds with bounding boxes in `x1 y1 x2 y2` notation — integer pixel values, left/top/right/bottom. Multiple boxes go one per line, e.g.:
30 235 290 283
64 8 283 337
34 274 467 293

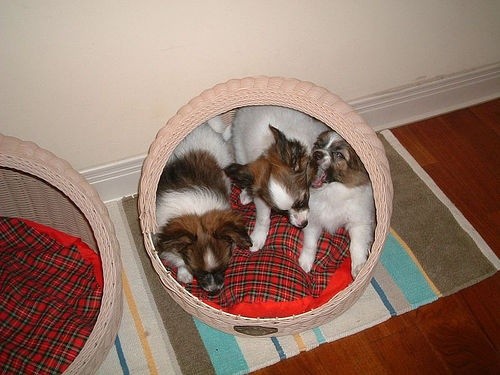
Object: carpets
83 126 500 375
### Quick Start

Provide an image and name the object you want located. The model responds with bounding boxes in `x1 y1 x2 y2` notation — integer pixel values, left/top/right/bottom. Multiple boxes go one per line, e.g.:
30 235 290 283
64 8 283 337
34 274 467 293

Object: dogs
222 104 312 252
298 130 375 280
155 117 253 293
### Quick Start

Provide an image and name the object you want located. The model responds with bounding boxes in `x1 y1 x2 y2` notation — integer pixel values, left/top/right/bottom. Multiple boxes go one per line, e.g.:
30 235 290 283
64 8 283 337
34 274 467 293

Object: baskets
137 76 395 339
0 133 124 375
